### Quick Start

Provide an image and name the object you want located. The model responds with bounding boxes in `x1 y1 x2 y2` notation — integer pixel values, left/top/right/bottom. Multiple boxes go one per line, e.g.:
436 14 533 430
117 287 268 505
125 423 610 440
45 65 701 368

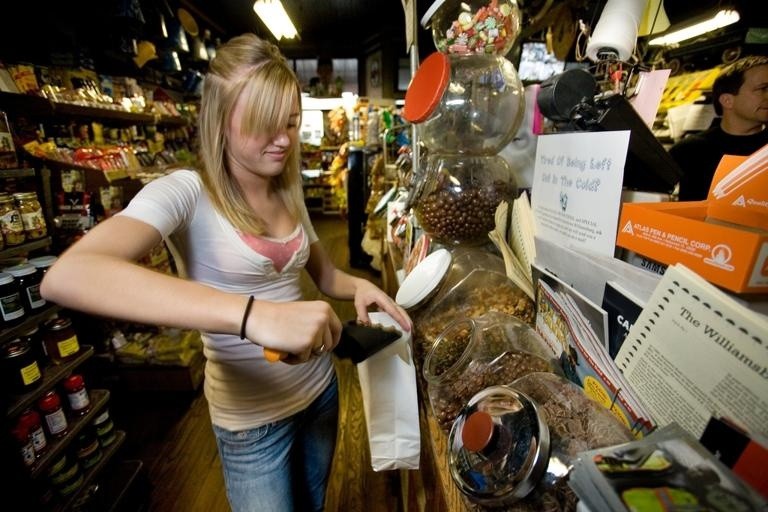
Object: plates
178 8 200 37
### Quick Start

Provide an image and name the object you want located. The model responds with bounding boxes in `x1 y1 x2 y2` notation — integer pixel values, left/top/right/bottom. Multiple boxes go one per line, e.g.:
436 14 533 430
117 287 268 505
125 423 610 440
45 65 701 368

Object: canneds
28 255 57 278
2 263 49 315
12 374 117 496
0 272 25 326
3 313 81 389
1 194 26 247
15 192 47 240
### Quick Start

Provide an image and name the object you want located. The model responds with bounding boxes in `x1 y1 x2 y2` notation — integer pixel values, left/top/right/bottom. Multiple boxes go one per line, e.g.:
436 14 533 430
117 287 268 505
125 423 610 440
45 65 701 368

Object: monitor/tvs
584 93 682 194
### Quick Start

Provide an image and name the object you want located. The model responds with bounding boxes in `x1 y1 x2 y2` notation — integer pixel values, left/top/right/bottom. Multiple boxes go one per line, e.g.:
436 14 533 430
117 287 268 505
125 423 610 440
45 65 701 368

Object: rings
314 345 325 355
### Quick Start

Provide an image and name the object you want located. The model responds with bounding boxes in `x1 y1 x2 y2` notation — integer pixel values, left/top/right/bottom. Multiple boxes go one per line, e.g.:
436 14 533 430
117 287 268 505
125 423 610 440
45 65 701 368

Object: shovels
263 320 403 366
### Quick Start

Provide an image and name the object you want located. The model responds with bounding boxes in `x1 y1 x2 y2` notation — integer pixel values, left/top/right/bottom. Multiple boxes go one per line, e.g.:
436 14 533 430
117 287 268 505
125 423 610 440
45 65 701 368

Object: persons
667 56 768 201
39 32 411 512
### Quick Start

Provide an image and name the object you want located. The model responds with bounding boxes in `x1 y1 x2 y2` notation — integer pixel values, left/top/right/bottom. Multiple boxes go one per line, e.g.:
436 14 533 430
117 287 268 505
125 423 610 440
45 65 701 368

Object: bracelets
240 296 254 339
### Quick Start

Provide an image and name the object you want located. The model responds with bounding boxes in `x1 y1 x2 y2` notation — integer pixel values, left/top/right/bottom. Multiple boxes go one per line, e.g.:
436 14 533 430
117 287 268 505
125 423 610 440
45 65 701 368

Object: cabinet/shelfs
1 89 217 512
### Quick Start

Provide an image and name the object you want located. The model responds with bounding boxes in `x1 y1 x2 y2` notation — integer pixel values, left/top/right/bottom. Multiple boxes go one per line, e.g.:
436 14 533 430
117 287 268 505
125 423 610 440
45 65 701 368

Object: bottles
34 71 114 105
422 313 568 440
409 153 519 247
420 1 524 59
402 50 526 157
393 247 534 366
320 156 328 170
443 372 634 511
353 117 360 142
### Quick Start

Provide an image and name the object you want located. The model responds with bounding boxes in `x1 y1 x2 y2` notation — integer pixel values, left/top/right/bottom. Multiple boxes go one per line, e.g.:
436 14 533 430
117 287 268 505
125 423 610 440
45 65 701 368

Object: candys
411 283 537 359
420 181 518 246
437 0 518 56
460 372 636 511
428 323 554 438
